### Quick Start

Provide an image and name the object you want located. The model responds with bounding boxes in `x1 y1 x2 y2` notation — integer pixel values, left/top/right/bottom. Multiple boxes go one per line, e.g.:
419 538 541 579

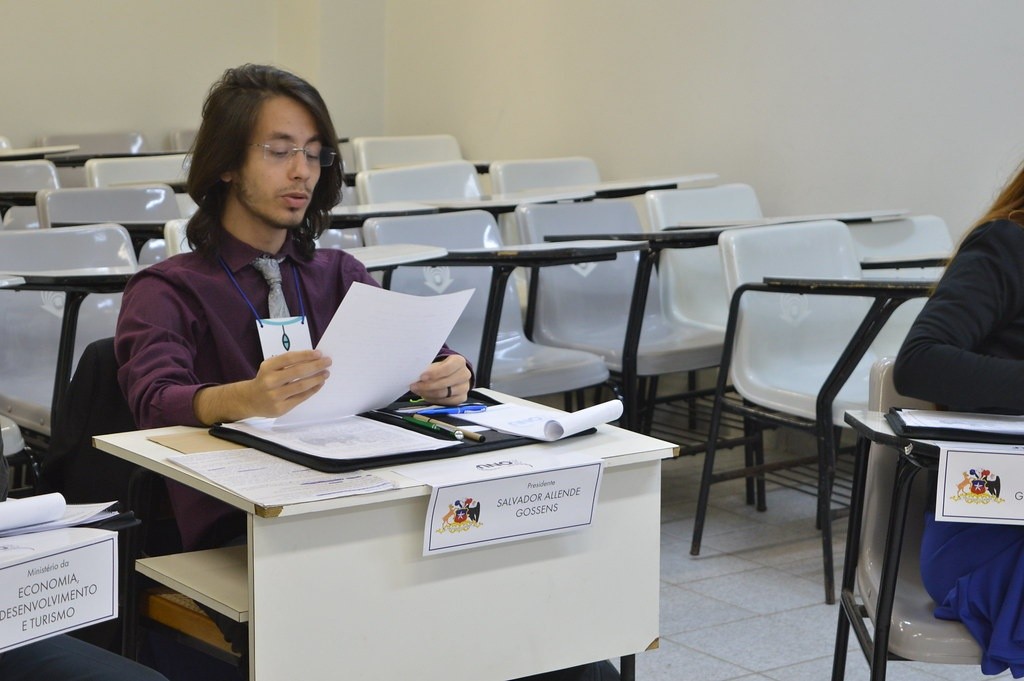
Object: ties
248 255 291 319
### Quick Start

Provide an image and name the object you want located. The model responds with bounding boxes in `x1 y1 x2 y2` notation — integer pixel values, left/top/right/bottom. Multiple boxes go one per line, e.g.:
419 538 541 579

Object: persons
894 159 1024 681
0 62 474 681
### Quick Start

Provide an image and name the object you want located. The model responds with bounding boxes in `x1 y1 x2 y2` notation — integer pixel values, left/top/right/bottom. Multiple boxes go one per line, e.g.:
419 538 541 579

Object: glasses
243 142 337 167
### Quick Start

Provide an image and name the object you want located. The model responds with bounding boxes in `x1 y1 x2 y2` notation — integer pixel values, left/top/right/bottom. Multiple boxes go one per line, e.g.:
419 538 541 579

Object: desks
658 208 906 226
50 219 166 259
542 226 739 434
403 188 598 222
92 386 681 681
0 143 80 161
685 274 938 604
326 203 441 229
572 172 718 204
400 238 649 390
0 264 153 446
860 251 954 271
345 243 448 290
844 410 1024 681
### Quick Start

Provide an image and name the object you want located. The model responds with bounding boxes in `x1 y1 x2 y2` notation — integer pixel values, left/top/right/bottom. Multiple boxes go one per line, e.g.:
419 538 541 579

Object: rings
447 386 452 398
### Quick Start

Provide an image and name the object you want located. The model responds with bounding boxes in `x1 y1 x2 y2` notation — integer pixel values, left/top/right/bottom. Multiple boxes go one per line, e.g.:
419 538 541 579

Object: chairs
512 197 767 514
360 209 610 412
354 158 481 206
0 219 139 491
33 181 180 230
831 356 983 681
488 155 601 284
643 182 780 504
28 336 243 661
42 130 149 188
0 158 60 229
351 133 462 171
850 212 955 354
690 220 883 529
85 152 193 189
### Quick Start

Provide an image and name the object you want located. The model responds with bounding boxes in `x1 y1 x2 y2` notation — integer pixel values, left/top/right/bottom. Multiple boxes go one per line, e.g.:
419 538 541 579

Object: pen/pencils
395 402 488 415
371 408 437 430
367 411 453 440
410 413 487 443
